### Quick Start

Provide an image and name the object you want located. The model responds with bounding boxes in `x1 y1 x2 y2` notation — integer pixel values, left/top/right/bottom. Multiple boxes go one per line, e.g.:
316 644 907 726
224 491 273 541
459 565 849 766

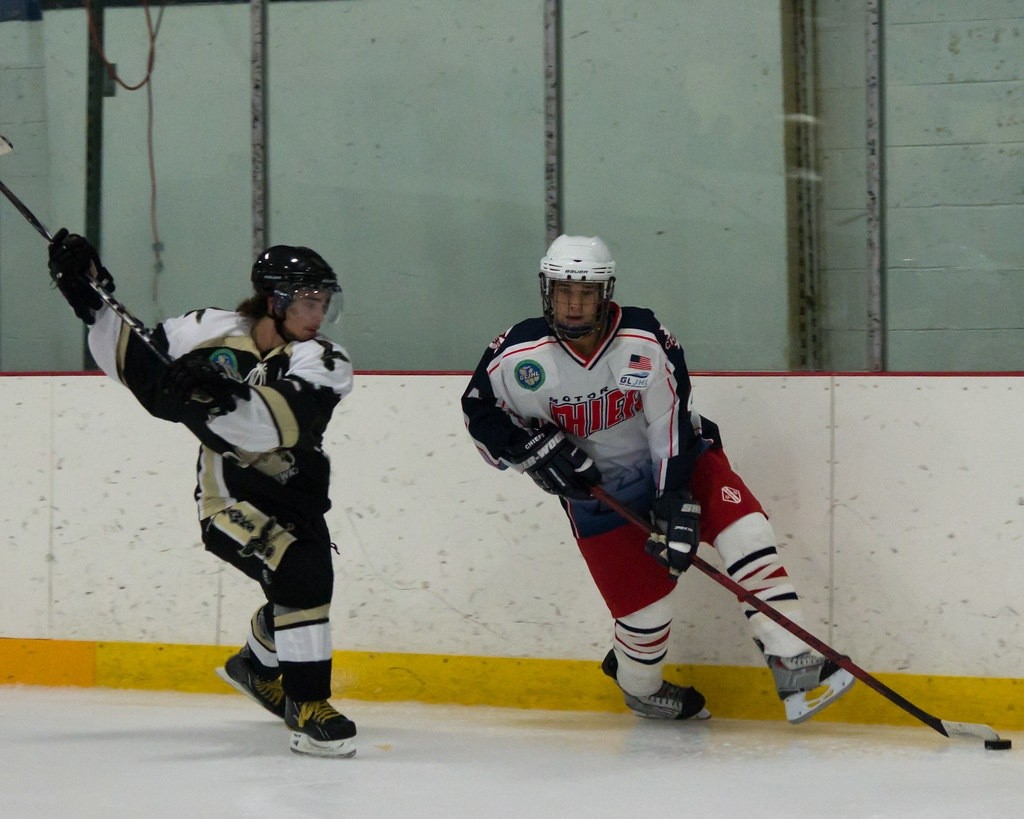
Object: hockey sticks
589 485 1014 754
0 134 175 371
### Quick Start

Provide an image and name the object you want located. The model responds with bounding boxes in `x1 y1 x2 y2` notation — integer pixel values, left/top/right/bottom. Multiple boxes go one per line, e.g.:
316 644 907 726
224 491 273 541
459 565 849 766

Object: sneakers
768 652 855 725
214 641 358 759
624 678 714 721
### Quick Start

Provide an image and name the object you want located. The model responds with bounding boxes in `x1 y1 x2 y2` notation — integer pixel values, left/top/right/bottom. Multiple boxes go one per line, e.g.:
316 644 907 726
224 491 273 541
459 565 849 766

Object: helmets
538 233 616 340
250 245 345 324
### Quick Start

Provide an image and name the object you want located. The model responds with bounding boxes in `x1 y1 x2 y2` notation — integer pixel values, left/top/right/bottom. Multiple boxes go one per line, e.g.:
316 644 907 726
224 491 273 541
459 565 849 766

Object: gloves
644 490 701 580
154 353 251 422
505 421 602 501
47 228 116 326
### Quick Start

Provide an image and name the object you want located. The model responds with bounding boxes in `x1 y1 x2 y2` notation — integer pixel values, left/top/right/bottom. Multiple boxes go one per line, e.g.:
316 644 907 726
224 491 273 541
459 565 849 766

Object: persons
47 230 357 760
461 234 855 724
